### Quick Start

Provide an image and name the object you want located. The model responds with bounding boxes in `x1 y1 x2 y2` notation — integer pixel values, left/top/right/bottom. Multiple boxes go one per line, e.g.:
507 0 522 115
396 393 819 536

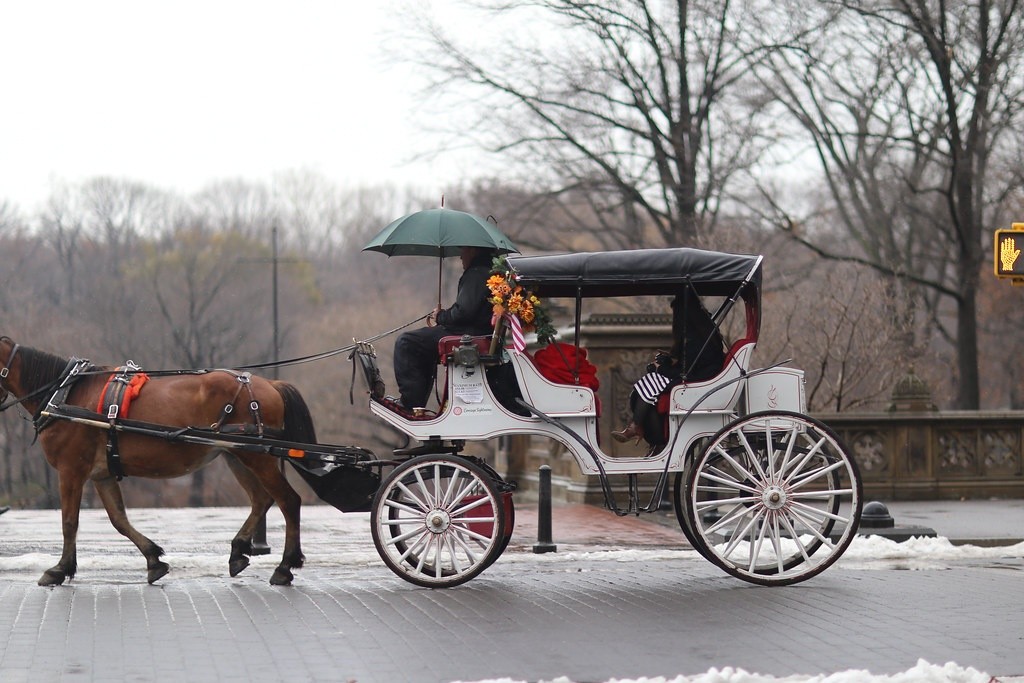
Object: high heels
644 444 667 457
610 422 646 447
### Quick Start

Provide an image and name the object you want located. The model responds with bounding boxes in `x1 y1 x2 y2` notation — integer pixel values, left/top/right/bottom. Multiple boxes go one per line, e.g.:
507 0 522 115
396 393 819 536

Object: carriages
0 247 865 589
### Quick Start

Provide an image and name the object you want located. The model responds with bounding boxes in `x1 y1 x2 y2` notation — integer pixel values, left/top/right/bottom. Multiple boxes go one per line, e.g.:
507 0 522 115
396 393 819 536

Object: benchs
437 277 508 392
651 338 757 448
505 342 605 423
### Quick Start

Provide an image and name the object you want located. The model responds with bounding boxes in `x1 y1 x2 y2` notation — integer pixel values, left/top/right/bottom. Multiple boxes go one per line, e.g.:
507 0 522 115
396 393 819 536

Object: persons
384 246 494 414
610 296 722 458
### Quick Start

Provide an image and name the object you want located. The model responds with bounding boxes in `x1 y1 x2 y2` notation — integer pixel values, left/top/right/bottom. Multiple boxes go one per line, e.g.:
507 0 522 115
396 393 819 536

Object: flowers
486 265 541 324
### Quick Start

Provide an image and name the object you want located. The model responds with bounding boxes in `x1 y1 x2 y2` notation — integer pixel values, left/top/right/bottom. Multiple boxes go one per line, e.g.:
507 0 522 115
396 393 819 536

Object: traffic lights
992 223 1024 281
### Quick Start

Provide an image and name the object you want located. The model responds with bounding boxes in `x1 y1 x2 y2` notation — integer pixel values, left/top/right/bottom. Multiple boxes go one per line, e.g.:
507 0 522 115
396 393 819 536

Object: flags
504 311 527 353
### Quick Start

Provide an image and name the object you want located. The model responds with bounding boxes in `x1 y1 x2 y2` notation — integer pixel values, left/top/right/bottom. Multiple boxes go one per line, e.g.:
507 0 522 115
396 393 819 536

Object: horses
0 334 326 587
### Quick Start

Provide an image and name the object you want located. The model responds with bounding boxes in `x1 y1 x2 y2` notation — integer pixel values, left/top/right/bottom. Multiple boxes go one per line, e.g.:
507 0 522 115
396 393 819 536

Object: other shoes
383 394 405 409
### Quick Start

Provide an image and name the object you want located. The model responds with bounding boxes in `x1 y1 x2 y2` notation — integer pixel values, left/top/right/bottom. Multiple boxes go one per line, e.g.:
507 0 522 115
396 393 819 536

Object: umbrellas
359 195 522 311
